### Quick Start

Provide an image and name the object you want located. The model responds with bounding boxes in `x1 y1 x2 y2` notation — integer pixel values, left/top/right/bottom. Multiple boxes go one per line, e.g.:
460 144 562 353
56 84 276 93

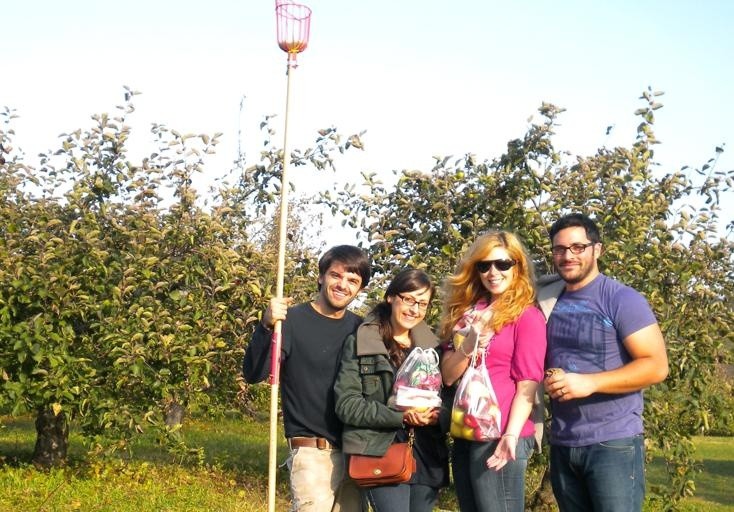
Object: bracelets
502 432 519 445
460 342 473 358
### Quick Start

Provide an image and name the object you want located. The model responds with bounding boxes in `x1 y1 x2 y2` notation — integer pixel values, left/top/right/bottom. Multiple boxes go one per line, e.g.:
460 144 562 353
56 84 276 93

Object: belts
286 436 326 450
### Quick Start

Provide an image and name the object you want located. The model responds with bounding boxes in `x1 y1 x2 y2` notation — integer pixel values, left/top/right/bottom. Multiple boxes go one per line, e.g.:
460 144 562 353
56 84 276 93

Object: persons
433 227 549 511
538 212 670 511
240 243 374 511
330 266 455 511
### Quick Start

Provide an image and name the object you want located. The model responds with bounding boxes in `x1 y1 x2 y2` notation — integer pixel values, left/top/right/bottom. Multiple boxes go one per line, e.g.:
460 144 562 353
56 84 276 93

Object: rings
560 388 564 394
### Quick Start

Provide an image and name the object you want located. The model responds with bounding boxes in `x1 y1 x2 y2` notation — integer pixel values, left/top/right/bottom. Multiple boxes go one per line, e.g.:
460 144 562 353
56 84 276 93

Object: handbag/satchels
347 441 414 488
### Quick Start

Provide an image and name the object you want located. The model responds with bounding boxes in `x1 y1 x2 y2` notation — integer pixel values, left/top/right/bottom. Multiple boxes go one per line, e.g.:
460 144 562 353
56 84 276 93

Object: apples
468 194 474 199
450 375 500 442
344 208 350 214
451 172 464 182
388 371 441 408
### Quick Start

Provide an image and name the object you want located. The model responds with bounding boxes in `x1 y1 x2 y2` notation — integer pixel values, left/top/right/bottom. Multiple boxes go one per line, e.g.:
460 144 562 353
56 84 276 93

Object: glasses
395 292 433 312
476 257 518 274
549 239 596 256
